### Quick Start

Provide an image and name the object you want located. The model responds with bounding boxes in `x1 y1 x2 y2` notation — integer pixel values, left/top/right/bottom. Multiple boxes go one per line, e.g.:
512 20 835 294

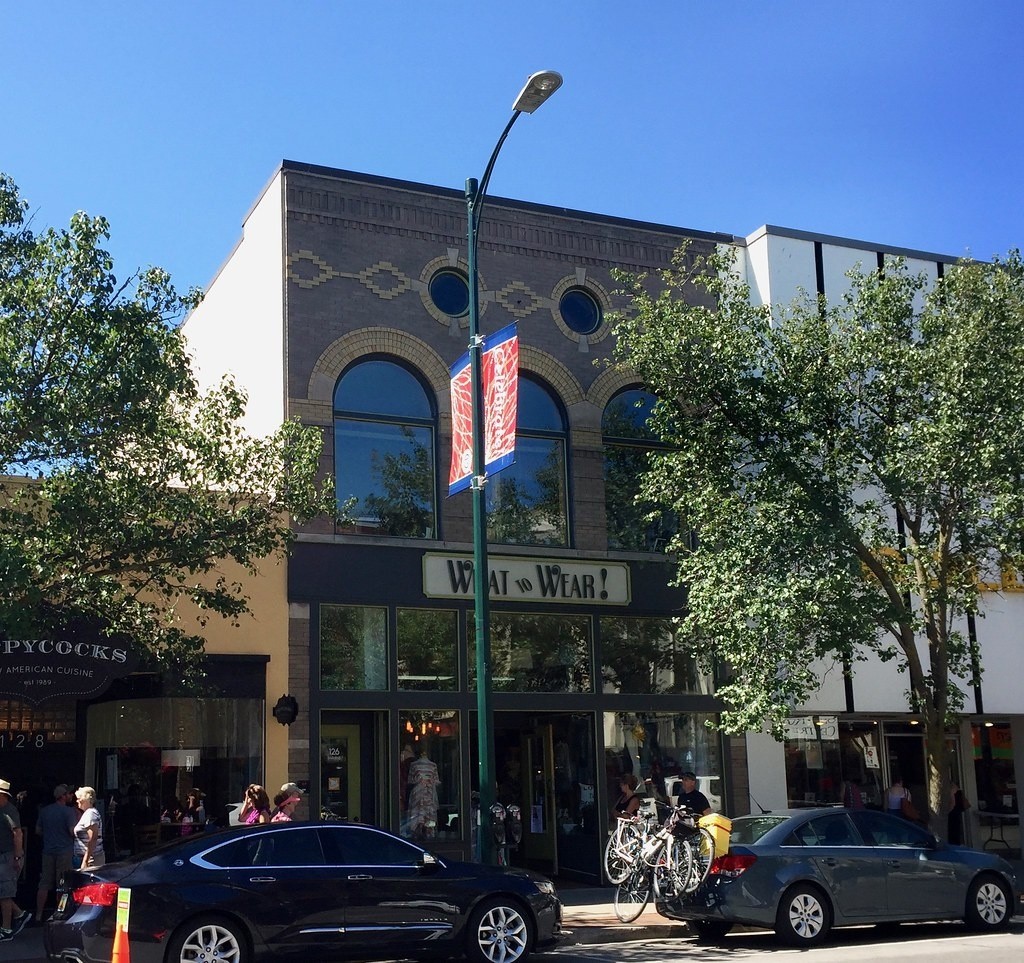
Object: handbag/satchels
954 790 970 813
901 787 918 820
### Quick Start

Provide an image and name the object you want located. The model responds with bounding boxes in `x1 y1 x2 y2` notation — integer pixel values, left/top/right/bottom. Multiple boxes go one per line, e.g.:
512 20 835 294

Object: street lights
459 64 570 891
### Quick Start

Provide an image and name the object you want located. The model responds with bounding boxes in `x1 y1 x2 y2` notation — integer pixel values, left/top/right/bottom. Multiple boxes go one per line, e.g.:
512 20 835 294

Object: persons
74 787 105 869
31 783 77 926
161 788 206 842
610 774 640 821
66 784 81 822
948 780 964 845
650 757 684 798
238 784 272 889
407 737 440 839
0 779 32 942
270 790 301 853
841 772 863 807
678 773 712 821
884 775 911 842
272 782 303 820
16 791 43 849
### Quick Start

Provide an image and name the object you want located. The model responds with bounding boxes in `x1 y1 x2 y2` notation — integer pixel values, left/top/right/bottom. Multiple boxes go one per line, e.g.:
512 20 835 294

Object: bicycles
603 795 717 923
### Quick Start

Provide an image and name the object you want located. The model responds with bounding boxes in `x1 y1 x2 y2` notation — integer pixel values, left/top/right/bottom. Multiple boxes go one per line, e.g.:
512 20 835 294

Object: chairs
816 820 851 846
132 823 160 851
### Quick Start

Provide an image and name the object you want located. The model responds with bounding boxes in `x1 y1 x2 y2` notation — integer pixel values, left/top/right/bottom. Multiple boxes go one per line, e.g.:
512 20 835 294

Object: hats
679 772 696 781
280 795 300 806
54 784 74 798
281 783 303 794
0 779 12 797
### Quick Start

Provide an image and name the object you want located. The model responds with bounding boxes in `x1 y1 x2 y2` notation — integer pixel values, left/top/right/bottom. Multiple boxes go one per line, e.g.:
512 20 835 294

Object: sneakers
13 911 32 935
0 927 13 942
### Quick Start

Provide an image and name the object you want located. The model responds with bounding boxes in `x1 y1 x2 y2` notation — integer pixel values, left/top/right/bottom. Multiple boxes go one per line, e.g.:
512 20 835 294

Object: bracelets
14 856 21 861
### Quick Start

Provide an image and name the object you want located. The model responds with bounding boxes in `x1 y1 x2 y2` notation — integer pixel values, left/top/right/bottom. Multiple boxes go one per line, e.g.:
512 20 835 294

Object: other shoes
33 920 42 928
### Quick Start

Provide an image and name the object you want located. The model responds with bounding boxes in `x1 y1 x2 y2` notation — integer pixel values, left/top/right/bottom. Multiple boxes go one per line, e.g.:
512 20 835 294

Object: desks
163 821 207 826
976 810 1021 857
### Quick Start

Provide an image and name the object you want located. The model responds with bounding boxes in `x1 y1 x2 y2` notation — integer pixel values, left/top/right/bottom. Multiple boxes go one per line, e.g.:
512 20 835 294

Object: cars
654 804 1021 947
43 821 563 962
